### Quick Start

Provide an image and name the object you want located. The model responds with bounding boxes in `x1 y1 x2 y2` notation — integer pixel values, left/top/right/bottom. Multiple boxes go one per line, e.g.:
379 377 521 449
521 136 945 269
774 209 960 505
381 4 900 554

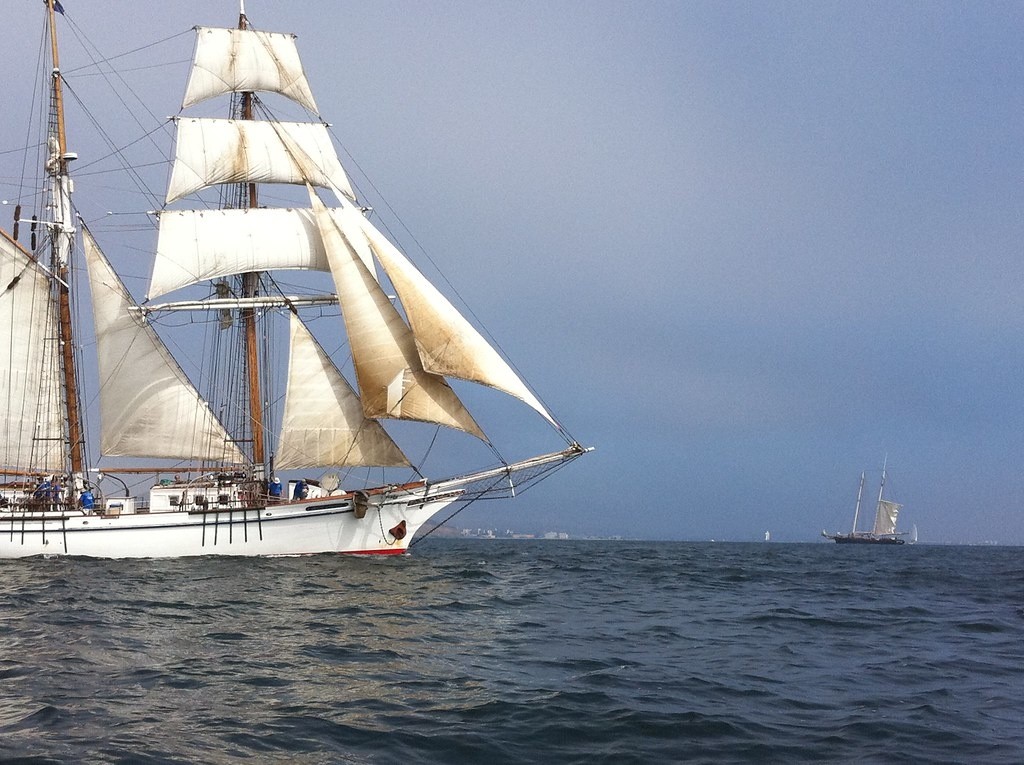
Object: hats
301 479 306 484
273 477 280 484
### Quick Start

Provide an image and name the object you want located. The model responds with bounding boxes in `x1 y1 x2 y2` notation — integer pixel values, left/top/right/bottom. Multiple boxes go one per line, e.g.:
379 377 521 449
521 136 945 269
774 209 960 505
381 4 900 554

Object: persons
266 477 284 499
79 487 95 515
33 478 60 501
293 478 309 501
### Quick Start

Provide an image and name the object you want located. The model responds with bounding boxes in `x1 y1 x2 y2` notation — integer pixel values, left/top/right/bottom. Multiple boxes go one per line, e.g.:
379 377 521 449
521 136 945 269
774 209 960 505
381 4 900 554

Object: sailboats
1 0 596 560
821 450 911 545
